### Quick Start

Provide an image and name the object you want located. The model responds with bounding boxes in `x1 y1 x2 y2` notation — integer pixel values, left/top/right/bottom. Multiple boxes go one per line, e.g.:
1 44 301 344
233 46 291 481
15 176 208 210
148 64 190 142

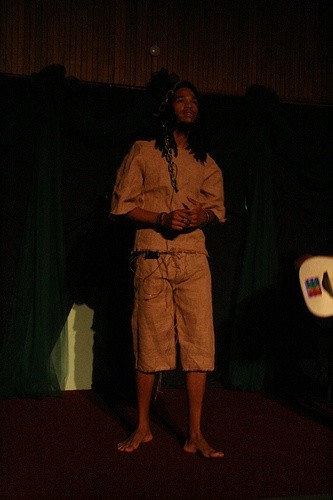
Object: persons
110 81 228 458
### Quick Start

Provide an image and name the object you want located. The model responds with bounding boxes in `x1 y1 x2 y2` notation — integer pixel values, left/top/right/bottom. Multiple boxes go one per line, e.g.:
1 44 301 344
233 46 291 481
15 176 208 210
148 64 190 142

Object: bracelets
202 210 209 228
156 212 166 227
